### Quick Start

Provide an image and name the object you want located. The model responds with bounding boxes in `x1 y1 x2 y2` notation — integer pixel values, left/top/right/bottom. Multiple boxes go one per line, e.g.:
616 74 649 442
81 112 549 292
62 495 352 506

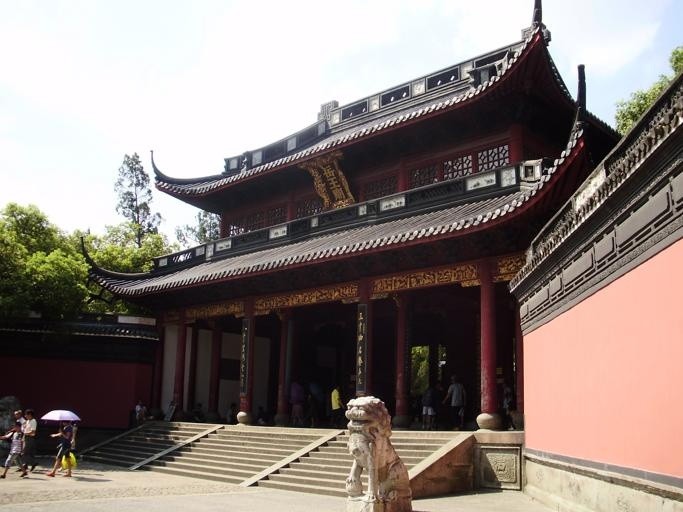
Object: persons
135 399 154 426
4 409 27 472
19 408 37 473
415 372 518 431
0 421 27 478
255 406 273 426
44 419 73 478
289 375 355 429
190 403 206 423
226 402 237 423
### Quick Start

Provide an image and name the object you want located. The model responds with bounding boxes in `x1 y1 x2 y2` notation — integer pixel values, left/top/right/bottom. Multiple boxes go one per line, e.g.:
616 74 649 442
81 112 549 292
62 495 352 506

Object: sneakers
46 471 56 478
63 473 71 477
0 474 5 479
58 467 62 472
18 463 37 478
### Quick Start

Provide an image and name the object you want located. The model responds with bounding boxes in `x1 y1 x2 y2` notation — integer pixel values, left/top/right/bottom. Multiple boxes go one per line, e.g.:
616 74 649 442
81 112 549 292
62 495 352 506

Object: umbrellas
39 408 82 435
